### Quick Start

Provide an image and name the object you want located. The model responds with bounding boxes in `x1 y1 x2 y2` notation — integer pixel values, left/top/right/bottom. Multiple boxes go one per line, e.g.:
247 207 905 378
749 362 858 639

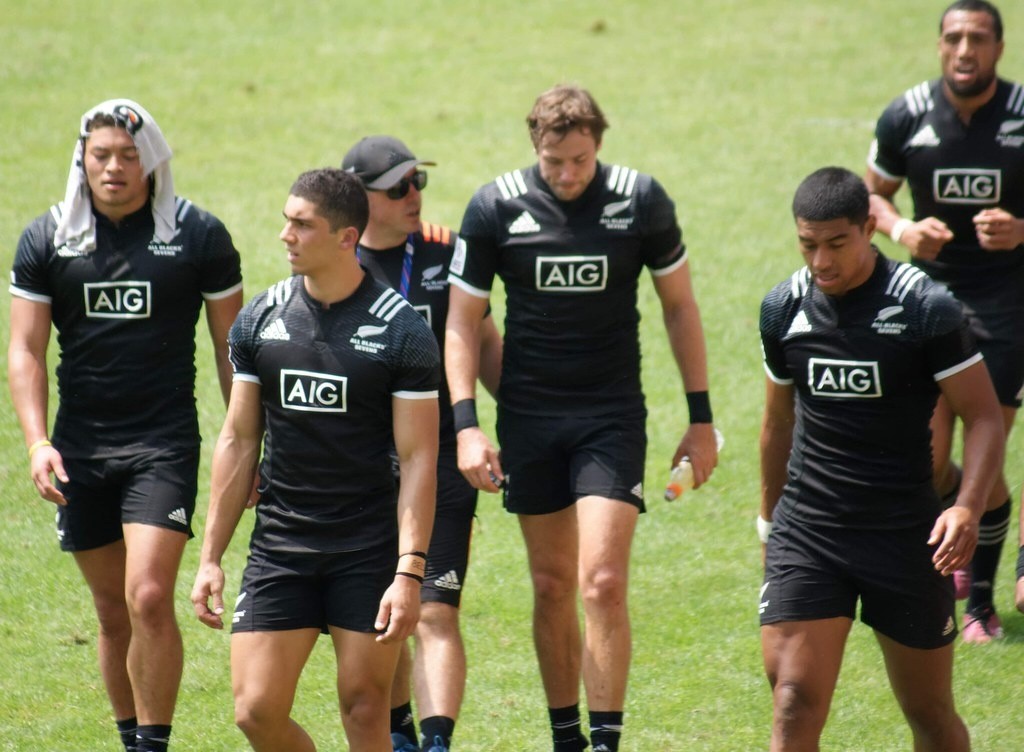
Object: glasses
388 171 427 198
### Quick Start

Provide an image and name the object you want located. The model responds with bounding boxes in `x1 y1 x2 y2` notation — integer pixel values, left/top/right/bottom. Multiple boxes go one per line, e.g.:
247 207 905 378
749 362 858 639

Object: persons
338 84 719 752
8 99 262 752
190 168 440 752
756 166 1006 752
864 0 1024 644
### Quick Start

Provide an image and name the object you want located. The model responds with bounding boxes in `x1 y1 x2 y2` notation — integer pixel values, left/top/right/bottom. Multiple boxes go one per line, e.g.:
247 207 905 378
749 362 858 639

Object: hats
342 134 438 190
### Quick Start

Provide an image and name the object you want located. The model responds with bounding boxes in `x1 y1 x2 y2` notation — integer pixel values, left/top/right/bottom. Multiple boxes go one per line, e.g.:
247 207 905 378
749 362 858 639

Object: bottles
666 431 723 501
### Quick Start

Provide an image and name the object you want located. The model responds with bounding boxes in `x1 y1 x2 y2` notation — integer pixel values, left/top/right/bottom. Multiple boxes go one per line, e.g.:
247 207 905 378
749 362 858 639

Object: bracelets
452 398 478 434
27 440 52 458
890 218 912 243
757 515 774 544
395 551 427 584
685 391 712 424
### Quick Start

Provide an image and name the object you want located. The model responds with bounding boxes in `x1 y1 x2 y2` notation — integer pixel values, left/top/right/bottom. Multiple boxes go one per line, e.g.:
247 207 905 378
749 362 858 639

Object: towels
54 98 176 253
